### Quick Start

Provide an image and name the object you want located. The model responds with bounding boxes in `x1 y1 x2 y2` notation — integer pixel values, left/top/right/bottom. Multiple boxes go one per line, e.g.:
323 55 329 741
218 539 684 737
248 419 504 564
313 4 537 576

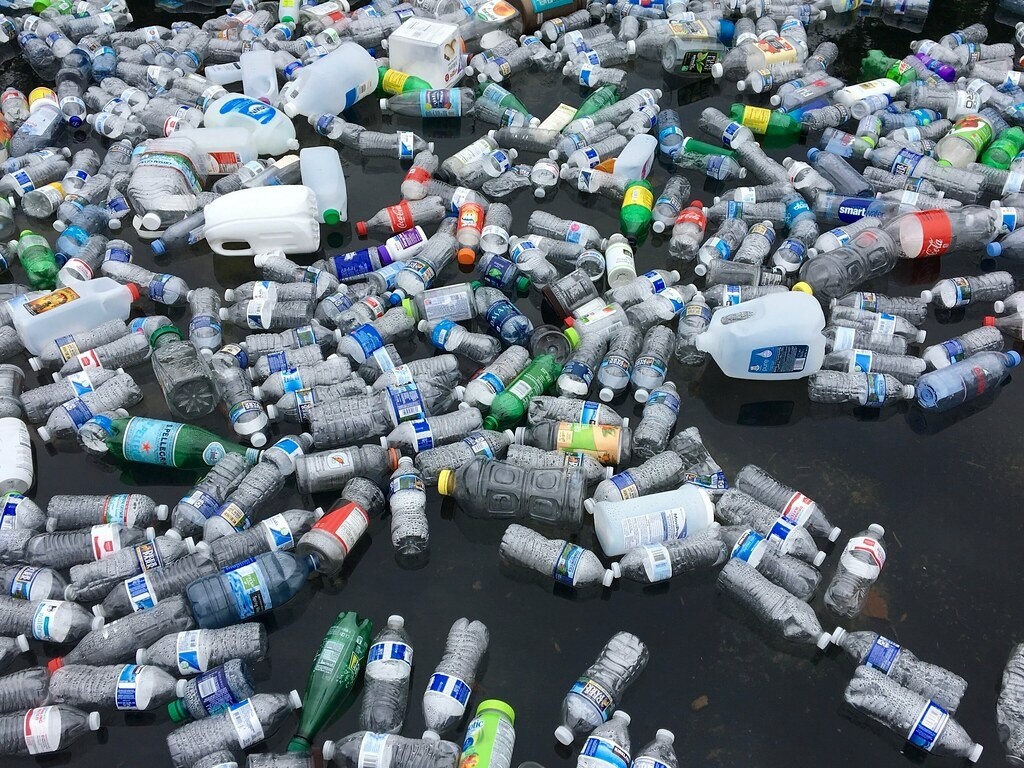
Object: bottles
2 0 1024 766
